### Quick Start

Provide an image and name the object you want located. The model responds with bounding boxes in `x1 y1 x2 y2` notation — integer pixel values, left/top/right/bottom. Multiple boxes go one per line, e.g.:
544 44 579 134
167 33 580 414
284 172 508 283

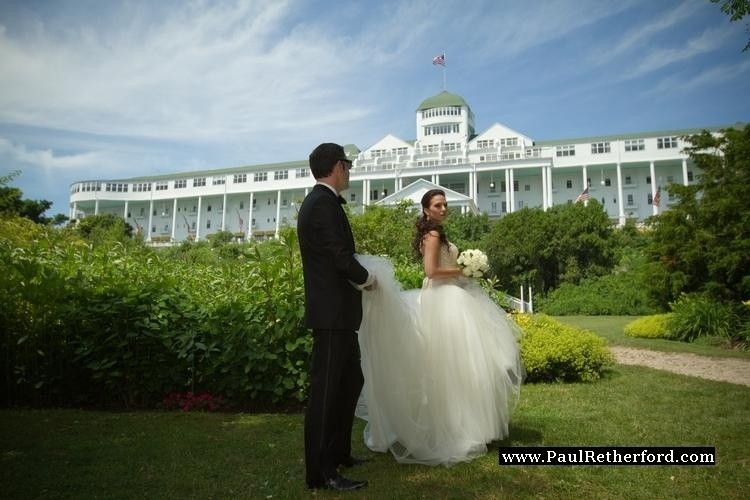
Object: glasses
338 158 352 169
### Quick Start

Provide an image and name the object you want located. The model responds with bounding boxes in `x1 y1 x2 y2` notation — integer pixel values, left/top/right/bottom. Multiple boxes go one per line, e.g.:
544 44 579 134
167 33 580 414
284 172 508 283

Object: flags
432 54 445 66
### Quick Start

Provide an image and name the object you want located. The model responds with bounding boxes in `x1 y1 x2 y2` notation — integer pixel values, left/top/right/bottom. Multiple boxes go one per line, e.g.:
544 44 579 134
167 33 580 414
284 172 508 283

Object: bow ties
337 194 347 205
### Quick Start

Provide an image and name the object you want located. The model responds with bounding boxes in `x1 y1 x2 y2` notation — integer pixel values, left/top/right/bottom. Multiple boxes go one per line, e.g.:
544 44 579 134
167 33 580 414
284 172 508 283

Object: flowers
457 249 491 278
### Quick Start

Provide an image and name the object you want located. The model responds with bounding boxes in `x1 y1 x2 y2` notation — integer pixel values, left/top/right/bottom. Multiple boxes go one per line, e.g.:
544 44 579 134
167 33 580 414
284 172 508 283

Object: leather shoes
326 471 369 492
339 454 369 468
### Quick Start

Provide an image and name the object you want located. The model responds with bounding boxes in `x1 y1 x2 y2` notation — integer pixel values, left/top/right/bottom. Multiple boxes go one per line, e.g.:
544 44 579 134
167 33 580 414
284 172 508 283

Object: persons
297 142 379 492
347 189 527 470
